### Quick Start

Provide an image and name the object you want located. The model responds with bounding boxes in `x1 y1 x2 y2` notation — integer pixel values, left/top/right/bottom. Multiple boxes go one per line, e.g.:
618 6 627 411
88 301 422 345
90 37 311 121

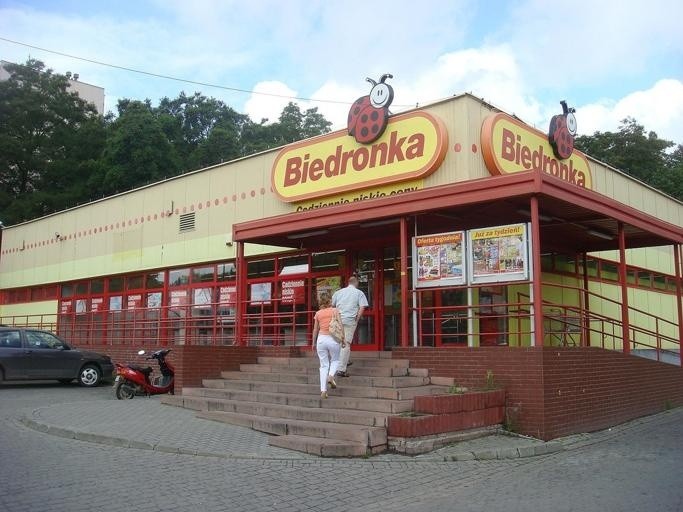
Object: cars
0 326 114 388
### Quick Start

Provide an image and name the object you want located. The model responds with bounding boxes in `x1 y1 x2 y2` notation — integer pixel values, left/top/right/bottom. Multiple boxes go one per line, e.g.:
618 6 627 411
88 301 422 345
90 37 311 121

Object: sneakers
337 371 349 377
321 392 329 398
328 377 336 389
348 360 352 366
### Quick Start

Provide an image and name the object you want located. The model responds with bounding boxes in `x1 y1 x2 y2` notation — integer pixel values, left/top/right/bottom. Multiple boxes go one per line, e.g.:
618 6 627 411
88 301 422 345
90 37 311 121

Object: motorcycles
112 347 173 401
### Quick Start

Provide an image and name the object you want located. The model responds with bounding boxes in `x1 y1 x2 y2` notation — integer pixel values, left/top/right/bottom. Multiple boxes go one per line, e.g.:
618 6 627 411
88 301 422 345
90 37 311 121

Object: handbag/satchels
328 319 343 343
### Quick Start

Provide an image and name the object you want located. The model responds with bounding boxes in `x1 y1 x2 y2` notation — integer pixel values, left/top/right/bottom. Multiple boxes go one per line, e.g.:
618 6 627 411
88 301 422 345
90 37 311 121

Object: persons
330 275 370 378
311 291 346 399
